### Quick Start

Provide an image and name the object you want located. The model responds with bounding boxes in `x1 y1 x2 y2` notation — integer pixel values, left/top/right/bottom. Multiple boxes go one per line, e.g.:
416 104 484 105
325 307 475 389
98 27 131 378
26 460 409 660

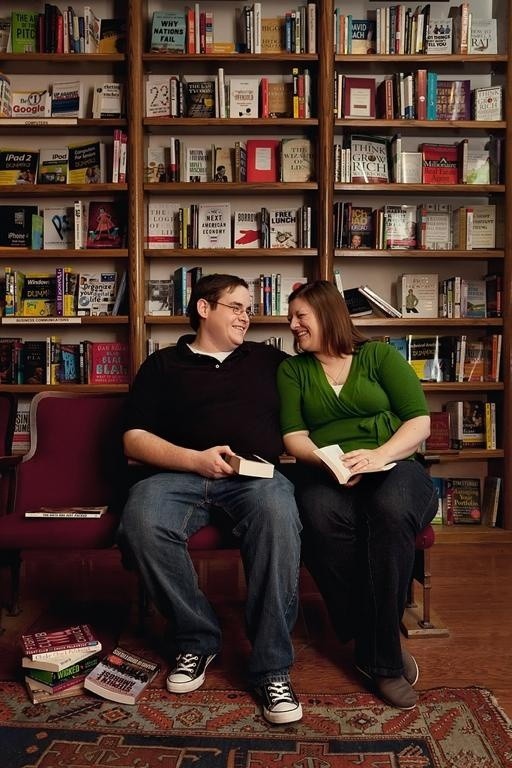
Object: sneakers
164 651 217 692
356 665 418 711
255 676 303 725
397 651 418 686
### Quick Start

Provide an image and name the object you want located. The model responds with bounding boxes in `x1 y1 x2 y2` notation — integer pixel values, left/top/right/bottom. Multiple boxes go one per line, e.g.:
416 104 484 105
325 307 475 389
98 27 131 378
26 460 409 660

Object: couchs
2 385 453 641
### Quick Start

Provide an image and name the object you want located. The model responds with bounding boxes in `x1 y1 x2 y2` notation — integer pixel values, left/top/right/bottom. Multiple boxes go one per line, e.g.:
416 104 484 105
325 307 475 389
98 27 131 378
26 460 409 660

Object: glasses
212 301 256 320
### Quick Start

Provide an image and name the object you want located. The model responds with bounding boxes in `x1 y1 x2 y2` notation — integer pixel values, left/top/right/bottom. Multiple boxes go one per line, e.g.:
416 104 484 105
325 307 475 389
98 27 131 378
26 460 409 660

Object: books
425 400 502 527
11 394 35 450
1 2 131 384
374 332 502 383
10 623 160 709
331 1 502 317
143 1 317 354
24 506 109 519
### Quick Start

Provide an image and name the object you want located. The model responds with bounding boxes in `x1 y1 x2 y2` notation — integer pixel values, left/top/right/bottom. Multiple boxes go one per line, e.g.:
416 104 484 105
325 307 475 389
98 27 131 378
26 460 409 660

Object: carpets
0 675 512 767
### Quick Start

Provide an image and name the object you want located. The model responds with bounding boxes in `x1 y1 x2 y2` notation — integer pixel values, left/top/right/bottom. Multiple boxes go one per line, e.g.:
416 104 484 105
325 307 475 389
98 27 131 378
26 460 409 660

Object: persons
277 279 441 710
115 272 304 724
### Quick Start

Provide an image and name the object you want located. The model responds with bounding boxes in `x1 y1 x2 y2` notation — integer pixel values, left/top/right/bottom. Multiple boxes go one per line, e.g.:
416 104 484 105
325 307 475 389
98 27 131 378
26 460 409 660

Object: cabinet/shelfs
140 0 328 391
328 0 511 542
1 1 138 454
